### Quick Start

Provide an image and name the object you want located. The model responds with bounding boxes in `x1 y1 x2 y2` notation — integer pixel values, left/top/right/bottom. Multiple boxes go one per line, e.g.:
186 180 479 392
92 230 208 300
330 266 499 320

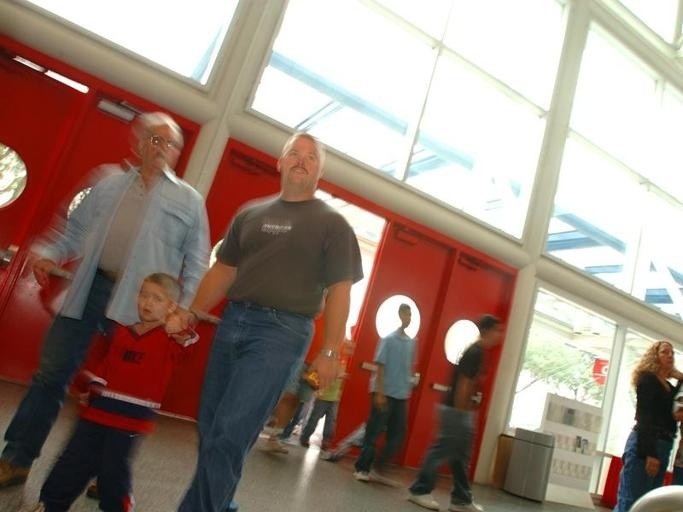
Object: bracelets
319 348 339 361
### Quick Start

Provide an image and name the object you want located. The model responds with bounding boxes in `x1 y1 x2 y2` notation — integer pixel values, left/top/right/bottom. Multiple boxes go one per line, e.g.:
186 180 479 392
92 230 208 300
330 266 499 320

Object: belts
98 268 116 282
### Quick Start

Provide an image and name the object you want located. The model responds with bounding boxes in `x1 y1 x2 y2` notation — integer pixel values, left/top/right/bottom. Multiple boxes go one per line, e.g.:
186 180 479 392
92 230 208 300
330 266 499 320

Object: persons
670 380 683 486
297 337 356 459
275 362 321 444
0 109 212 501
36 271 199 512
406 315 504 512
353 302 416 487
166 130 365 512
608 341 682 511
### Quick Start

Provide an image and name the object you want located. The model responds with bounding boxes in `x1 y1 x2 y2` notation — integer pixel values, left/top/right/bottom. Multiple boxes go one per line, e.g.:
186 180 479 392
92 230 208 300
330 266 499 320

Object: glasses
148 135 177 150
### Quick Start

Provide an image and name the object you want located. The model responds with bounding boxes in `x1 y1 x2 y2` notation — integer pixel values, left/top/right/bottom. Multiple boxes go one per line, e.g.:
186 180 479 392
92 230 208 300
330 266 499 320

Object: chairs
628 485 683 512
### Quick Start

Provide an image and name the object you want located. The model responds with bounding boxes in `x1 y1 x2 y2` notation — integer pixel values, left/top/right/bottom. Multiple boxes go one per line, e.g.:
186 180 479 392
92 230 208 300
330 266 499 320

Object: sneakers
87 479 100 499
448 498 482 511
409 491 440 509
0 459 30 487
300 437 310 448
353 469 369 480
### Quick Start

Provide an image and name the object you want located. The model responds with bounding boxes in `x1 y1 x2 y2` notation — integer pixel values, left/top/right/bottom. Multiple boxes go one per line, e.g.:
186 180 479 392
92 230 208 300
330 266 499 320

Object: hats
478 315 502 328
